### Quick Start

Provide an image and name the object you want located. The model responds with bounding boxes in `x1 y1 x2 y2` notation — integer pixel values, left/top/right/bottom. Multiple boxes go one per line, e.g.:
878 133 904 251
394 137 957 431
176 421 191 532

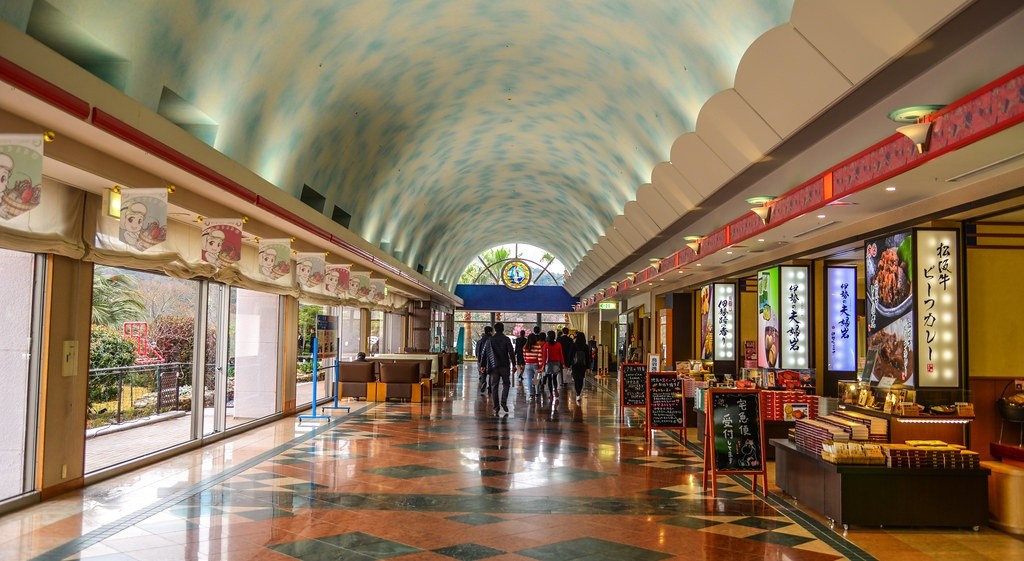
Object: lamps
572 276 635 310
650 262 660 273
686 242 699 255
102 186 122 220
751 207 771 224
896 117 931 153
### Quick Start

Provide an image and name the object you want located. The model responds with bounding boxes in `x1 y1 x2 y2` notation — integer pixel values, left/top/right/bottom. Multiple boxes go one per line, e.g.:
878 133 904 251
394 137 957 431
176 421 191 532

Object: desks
760 420 991 533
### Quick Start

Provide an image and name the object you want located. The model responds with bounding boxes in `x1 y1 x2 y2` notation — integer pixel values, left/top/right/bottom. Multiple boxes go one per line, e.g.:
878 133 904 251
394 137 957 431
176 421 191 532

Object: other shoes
493 407 498 413
548 396 553 401
554 389 558 397
519 375 523 379
577 396 581 401
501 403 509 412
488 386 491 393
481 382 487 393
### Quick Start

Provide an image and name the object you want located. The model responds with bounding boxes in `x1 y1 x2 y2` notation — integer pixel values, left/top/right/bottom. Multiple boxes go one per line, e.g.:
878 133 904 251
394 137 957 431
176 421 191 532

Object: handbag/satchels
562 369 573 383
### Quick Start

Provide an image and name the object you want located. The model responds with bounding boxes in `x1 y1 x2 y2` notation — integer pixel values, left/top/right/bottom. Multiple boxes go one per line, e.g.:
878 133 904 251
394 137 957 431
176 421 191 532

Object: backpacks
575 350 586 366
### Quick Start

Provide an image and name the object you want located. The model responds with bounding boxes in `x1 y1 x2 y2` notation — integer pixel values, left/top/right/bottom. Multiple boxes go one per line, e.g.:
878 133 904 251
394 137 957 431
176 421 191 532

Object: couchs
335 360 376 401
376 350 458 403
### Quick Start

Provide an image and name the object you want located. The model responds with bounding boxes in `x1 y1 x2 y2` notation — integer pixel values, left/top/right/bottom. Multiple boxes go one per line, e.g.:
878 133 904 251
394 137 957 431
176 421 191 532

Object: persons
474 322 599 413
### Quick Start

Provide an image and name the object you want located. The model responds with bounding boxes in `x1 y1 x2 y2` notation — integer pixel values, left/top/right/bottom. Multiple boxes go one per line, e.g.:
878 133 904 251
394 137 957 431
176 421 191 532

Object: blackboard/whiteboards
618 364 646 408
645 371 686 429
708 388 766 475
